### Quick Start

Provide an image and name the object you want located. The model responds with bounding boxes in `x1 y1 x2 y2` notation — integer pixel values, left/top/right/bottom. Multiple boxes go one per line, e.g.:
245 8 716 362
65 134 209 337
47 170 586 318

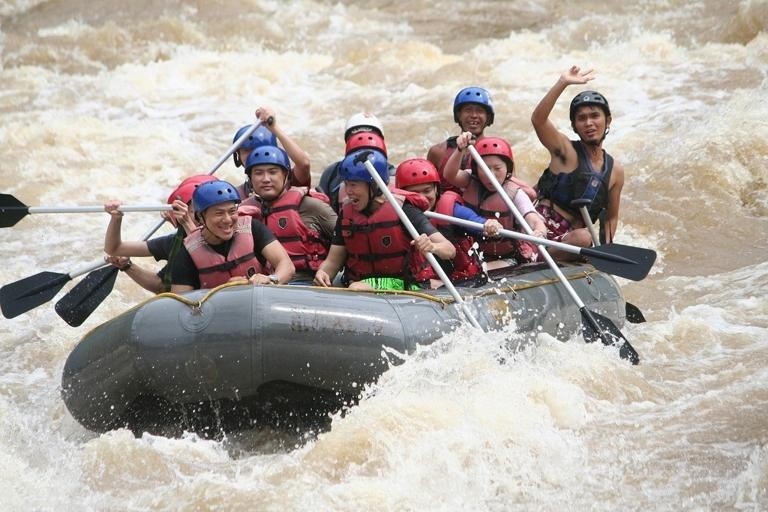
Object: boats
61 258 626 435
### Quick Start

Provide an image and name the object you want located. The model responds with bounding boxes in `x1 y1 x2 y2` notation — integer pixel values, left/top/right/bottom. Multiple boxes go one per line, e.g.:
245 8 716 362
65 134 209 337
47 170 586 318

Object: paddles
572 199 646 323
0 253 111 318
57 114 274 327
459 134 640 368
0 192 183 227
421 208 657 282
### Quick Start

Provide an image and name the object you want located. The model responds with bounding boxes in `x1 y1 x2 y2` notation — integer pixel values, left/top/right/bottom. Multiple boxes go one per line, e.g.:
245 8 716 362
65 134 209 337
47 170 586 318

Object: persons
105 64 626 297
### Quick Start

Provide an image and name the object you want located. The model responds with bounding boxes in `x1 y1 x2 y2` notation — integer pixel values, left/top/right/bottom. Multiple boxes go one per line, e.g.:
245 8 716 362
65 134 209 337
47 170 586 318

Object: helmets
245 147 290 178
453 87 495 125
346 130 387 154
395 158 443 190
340 149 389 196
345 112 384 135
233 124 278 150
469 136 514 172
569 90 611 133
165 183 197 205
176 175 218 186
192 179 240 223
